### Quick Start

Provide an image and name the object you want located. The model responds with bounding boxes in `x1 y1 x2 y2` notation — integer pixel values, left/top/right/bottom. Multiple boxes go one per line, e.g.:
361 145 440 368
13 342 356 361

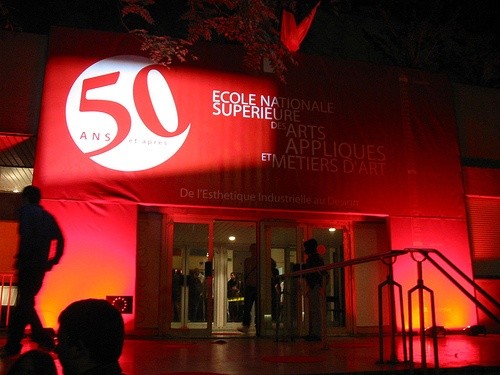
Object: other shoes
238 326 248 333
304 335 319 340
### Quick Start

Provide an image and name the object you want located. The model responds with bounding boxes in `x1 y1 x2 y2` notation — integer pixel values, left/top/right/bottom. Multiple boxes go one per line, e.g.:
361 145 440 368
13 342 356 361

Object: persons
0 185 63 357
57 299 125 375
237 243 257 332
173 267 186 323
270 257 281 322
228 272 243 322
8 350 56 375
303 238 323 342
200 273 214 322
187 268 205 321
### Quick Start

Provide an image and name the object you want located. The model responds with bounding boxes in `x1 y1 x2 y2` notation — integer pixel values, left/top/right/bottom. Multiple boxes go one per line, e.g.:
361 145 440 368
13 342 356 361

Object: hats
304 239 318 251
